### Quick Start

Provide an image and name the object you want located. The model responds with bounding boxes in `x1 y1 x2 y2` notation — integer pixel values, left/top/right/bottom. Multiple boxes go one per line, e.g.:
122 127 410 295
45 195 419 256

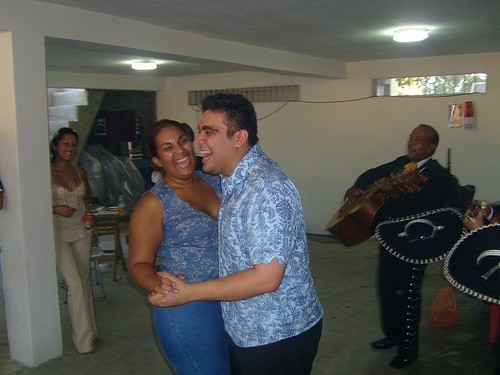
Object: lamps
131 58 158 71
392 27 430 43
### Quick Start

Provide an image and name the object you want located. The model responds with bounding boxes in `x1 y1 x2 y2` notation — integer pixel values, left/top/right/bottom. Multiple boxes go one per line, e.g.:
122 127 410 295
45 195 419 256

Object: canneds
84 213 93 229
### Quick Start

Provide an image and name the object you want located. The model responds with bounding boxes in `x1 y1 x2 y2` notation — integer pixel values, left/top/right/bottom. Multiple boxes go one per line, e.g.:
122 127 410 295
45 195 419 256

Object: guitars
324 161 428 248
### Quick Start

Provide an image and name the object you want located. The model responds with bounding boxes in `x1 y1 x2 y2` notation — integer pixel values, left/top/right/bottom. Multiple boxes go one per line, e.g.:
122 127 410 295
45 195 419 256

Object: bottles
117 195 126 215
84 204 95 230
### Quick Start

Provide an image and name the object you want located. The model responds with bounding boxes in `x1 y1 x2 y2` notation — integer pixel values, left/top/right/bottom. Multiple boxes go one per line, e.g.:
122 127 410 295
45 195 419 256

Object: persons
124 119 231 375
345 124 459 369
51 127 99 354
148 94 324 375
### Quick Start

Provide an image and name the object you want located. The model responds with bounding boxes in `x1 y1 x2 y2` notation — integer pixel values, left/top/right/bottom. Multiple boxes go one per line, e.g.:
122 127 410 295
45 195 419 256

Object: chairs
91 218 130 282
62 245 107 303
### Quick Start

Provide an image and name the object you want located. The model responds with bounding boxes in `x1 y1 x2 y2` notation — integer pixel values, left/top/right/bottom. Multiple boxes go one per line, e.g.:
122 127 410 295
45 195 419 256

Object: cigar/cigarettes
73 210 79 214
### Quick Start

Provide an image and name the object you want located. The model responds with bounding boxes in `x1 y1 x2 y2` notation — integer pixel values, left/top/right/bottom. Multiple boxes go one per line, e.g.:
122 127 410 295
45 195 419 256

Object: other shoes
388 351 419 369
370 336 401 350
